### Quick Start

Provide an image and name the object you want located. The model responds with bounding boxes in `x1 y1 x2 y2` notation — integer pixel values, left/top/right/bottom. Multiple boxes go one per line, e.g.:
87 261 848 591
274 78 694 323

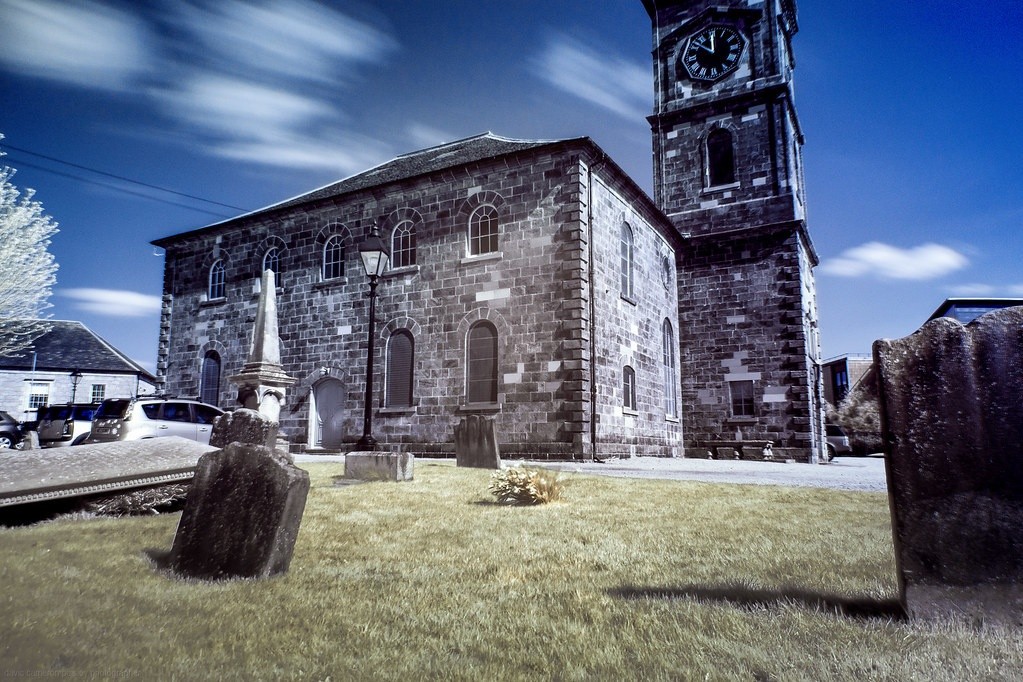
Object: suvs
36 394 228 445
825 423 854 463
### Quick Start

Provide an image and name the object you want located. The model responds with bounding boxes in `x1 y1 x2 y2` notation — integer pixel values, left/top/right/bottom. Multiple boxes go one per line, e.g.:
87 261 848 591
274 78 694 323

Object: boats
0 436 230 506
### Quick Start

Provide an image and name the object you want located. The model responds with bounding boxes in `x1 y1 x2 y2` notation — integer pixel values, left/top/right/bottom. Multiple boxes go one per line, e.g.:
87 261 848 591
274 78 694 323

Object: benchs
704 438 776 460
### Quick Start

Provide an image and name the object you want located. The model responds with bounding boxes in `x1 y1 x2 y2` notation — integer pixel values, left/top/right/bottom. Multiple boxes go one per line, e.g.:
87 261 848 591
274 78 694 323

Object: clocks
679 21 745 82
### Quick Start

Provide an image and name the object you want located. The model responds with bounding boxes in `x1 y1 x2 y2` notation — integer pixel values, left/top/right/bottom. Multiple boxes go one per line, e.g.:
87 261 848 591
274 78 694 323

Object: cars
0 411 23 450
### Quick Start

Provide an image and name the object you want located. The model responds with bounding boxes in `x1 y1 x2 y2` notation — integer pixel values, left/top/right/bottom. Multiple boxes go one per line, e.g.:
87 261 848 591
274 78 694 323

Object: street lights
68 368 83 403
355 219 391 452
